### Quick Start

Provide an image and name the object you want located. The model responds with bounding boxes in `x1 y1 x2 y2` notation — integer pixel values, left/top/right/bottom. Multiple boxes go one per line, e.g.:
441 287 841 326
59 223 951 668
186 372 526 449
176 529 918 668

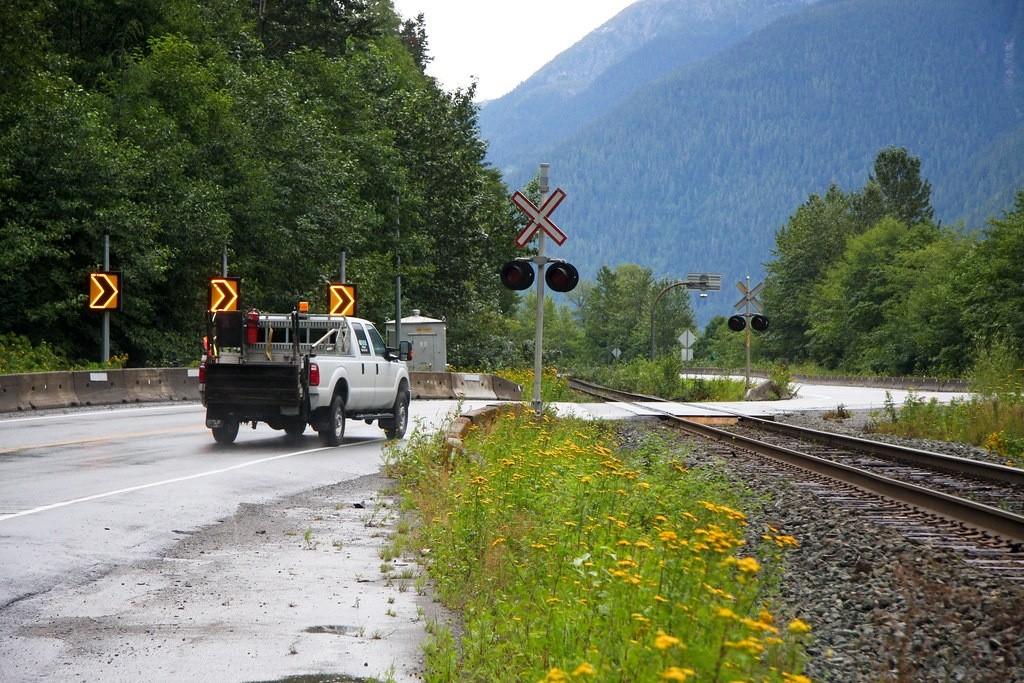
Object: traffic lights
728 316 746 331
544 263 579 294
501 261 534 290
751 315 769 331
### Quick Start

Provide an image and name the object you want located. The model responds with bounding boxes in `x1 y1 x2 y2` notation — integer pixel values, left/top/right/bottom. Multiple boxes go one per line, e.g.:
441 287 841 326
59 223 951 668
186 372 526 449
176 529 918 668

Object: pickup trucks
198 302 414 447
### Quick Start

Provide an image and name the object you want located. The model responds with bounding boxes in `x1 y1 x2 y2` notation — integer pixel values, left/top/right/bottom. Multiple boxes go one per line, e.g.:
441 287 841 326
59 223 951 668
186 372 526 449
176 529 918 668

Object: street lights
651 281 708 362
